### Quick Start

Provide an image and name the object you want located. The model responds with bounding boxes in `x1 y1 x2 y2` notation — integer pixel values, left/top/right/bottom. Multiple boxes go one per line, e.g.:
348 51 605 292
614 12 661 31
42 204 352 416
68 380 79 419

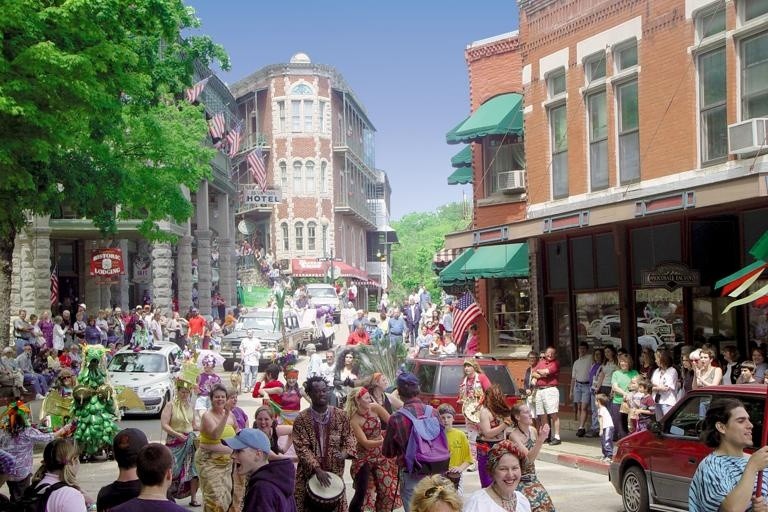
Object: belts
538 385 556 389
575 380 591 384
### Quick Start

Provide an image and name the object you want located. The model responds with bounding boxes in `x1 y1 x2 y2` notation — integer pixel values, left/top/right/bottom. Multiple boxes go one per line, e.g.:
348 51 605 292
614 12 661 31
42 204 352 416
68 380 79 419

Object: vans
304 282 341 325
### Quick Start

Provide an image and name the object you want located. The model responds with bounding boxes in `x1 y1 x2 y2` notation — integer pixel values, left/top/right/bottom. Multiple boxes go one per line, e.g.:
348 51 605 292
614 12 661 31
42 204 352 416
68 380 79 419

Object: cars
401 359 516 428
607 382 764 506
105 340 187 418
595 318 653 346
295 308 335 351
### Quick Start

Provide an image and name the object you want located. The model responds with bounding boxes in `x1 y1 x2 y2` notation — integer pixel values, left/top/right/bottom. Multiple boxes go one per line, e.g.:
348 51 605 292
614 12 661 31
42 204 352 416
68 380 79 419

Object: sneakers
466 463 478 472
35 394 45 400
243 387 249 393
575 427 586 436
250 387 254 393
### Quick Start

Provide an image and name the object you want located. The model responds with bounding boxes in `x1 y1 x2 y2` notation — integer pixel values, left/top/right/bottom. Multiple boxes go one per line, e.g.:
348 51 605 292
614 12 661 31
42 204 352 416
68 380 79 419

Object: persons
409 475 464 512
194 384 246 512
509 403 556 512
162 380 202 507
224 387 248 431
0 449 17 512
377 299 388 320
388 310 404 348
144 252 242 350
343 301 357 335
351 309 370 334
451 289 484 346
382 372 451 512
306 343 324 380
0 304 144 401
689 398 768 512
293 376 357 512
14 439 97 512
0 415 73 501
346 323 370 349
97 428 149 512
681 346 694 392
690 347 703 367
220 429 298 512
253 405 293 456
650 349 678 421
619 375 644 438
464 324 480 354
476 384 514 489
465 440 532 512
108 443 193 512
348 386 404 512
239 330 262 393
531 347 561 445
539 351 547 359
335 281 341 295
332 349 359 410
641 347 660 382
316 347 338 409
195 395 212 450
658 344 684 393
635 382 656 433
741 361 760 384
524 351 541 437
377 313 389 336
340 282 347 308
595 393 614 463
456 358 491 472
194 354 222 397
281 369 312 425
569 341 595 438
401 284 457 357
752 346 768 383
364 317 384 344
702 343 722 369
722 345 740 384
611 353 639 440
593 344 620 402
585 349 605 438
691 348 722 418
354 373 403 430
437 403 474 491
251 364 282 409
348 282 357 305
243 240 312 323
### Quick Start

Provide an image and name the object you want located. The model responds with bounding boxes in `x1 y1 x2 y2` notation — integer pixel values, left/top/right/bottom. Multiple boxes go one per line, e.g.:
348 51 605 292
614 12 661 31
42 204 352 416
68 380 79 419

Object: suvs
220 310 304 372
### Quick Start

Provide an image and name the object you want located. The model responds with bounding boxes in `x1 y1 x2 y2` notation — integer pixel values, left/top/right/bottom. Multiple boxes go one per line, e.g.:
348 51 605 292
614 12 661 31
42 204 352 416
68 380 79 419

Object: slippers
190 500 202 507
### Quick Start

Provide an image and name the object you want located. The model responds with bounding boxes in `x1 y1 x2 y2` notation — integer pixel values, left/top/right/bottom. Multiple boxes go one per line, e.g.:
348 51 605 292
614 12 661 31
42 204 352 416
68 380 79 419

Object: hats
78 304 87 310
112 428 149 458
396 373 420 387
143 305 150 309
136 305 142 309
221 428 271 452
366 318 380 326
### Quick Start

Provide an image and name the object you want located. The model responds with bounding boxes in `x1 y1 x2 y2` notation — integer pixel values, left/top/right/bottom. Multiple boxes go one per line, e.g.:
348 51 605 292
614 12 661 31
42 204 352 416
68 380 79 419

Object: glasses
425 486 448 499
690 360 699 362
539 356 546 359
62 375 72 380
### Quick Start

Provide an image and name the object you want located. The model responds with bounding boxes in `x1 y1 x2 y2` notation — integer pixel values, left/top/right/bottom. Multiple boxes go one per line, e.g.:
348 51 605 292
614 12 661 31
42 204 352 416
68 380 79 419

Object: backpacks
14 480 70 512
397 404 451 481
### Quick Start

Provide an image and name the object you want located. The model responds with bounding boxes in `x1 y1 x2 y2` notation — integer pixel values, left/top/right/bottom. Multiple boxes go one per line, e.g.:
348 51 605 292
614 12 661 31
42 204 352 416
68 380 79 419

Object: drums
306 472 346 512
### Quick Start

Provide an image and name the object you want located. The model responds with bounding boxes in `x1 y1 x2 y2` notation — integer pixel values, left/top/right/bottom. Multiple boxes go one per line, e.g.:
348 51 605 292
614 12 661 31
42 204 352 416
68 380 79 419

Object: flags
207 108 226 138
50 263 58 310
225 121 243 158
248 146 269 194
187 78 208 104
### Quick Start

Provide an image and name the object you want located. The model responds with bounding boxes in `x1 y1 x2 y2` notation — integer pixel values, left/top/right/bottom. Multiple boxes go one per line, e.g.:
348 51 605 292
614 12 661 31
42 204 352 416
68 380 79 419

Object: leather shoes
17 386 28 392
549 437 562 445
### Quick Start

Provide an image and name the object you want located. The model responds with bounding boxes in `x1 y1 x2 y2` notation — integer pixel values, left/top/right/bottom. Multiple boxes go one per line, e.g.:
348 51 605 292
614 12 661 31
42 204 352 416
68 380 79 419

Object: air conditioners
727 117 768 155
498 171 526 191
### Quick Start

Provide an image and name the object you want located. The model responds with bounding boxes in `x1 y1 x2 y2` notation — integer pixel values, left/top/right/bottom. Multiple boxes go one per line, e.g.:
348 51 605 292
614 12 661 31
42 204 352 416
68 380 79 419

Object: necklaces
489 485 518 510
